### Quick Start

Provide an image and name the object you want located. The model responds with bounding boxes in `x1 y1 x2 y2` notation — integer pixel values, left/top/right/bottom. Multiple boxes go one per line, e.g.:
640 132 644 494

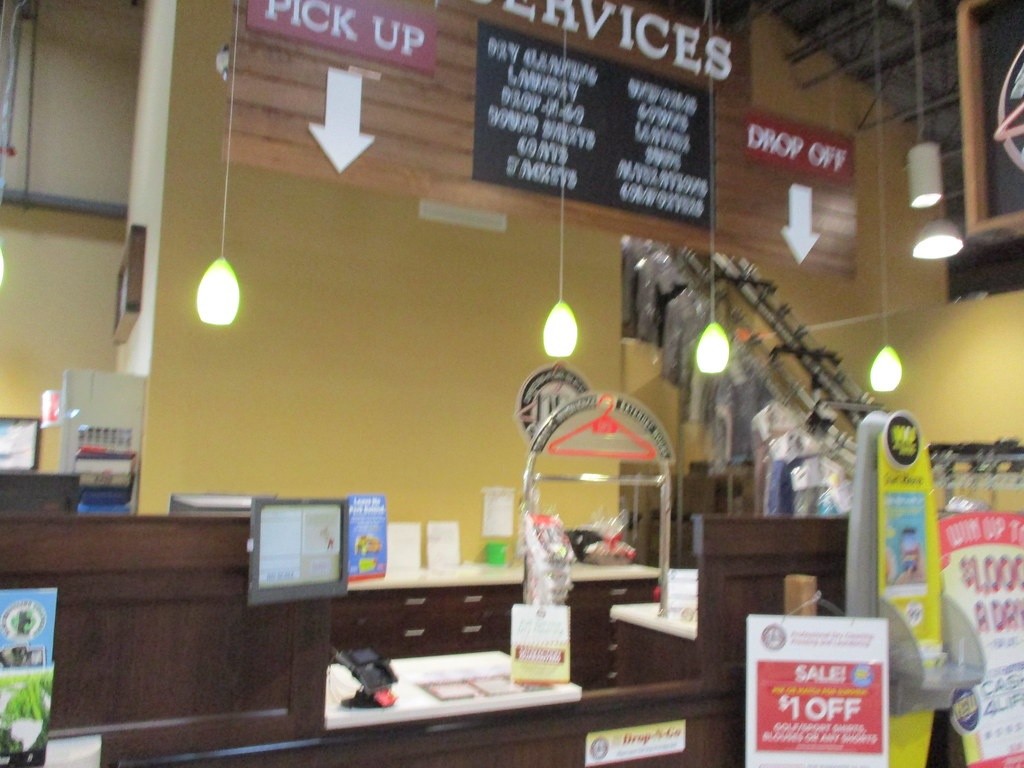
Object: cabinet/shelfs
0 505 850 768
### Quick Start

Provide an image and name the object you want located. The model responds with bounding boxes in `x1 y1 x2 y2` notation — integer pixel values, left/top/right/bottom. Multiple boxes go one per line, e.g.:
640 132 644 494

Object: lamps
693 0 729 376
912 220 966 262
196 1 241 327
541 3 580 359
904 141 946 211
867 1 905 394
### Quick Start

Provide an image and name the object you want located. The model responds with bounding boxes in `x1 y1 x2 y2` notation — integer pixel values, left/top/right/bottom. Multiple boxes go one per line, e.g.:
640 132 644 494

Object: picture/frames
111 224 147 342
